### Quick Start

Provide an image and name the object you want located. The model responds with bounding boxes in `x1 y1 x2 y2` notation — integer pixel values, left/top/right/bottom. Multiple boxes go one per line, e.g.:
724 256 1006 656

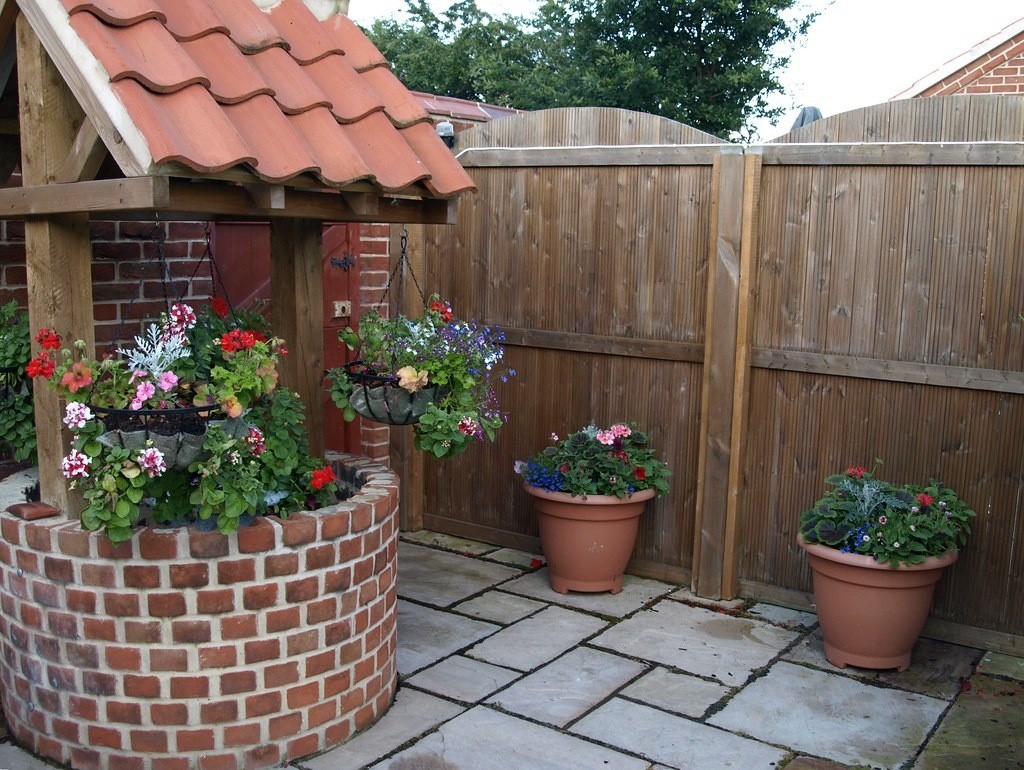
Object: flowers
513 419 670 498
795 459 972 564
23 292 325 529
326 301 515 462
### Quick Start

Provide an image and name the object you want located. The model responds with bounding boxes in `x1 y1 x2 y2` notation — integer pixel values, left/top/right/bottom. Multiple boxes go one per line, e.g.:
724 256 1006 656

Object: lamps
436 121 455 149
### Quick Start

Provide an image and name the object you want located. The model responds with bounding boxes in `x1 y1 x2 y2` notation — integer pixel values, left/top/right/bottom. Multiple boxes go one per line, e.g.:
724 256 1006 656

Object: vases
100 382 257 475
525 483 660 598
790 532 961 671
342 366 440 430
0 449 405 770
